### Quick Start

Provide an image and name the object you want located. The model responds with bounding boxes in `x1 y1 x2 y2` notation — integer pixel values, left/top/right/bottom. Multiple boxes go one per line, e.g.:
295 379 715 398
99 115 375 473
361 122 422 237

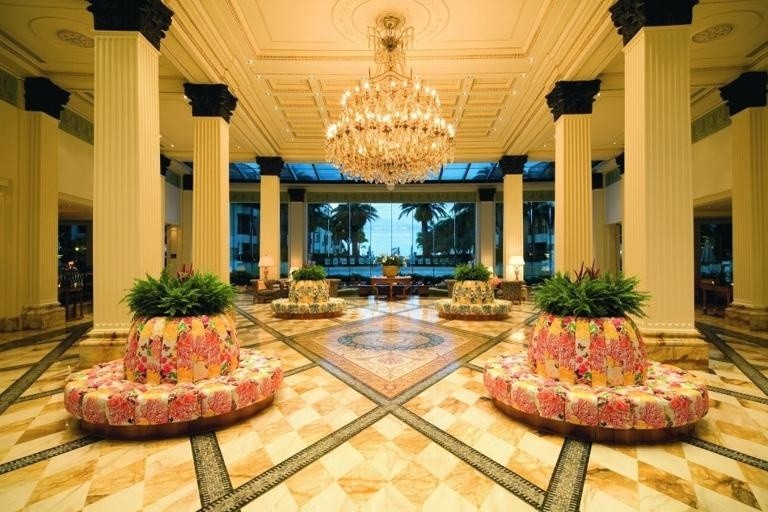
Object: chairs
251 281 277 302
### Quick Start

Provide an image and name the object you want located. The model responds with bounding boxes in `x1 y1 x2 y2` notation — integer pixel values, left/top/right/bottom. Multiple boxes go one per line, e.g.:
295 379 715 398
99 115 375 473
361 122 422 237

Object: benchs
356 285 430 297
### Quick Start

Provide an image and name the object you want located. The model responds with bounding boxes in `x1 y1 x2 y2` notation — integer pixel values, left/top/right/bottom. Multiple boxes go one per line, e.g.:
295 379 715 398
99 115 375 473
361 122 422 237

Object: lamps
323 11 455 191
509 256 525 282
258 256 274 280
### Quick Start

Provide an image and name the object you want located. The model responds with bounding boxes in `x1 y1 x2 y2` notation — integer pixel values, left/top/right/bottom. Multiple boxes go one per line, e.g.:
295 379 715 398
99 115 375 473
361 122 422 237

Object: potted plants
381 254 404 278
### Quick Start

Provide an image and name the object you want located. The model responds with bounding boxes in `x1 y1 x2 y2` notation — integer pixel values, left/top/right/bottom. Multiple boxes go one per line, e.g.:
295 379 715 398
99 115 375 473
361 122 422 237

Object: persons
68 260 84 288
61 264 73 306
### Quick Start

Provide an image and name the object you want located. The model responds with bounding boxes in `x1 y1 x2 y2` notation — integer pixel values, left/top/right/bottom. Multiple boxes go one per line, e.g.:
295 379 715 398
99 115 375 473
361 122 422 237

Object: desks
519 283 527 300
60 289 84 322
701 283 734 315
371 276 412 301
